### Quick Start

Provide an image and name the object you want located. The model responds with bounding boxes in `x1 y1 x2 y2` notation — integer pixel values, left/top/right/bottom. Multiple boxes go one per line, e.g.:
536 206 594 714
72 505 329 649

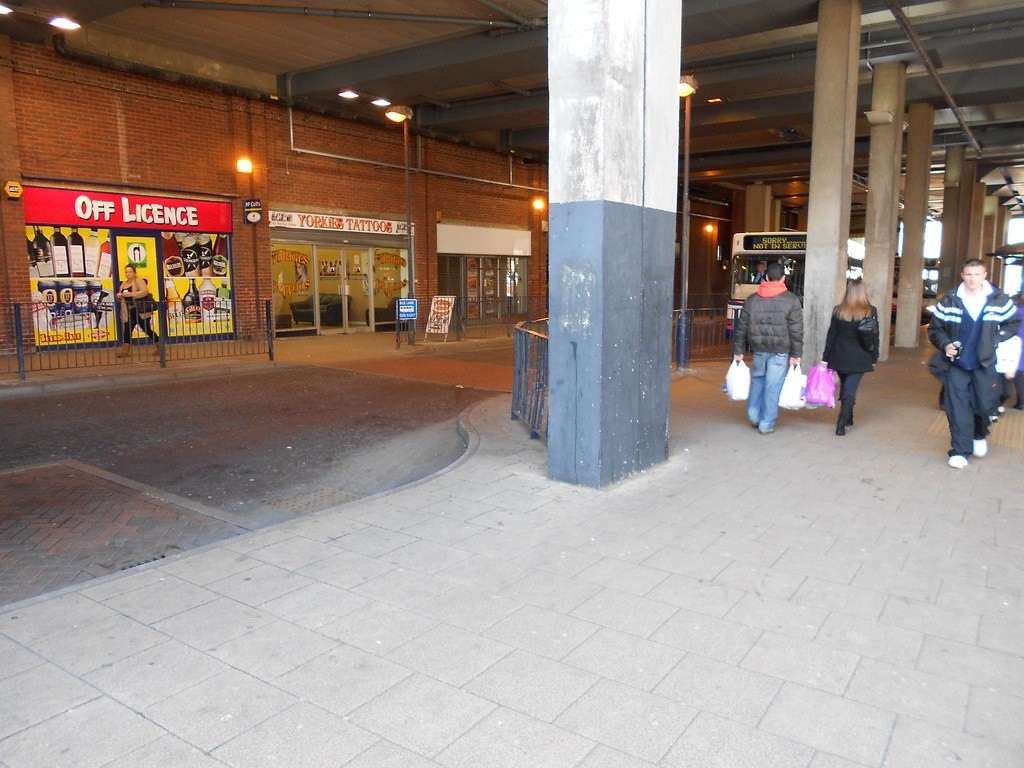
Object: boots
153 341 161 356
117 343 130 357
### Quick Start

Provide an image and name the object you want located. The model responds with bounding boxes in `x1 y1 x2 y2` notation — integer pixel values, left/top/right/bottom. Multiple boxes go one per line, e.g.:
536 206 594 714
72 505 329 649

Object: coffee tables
274 314 292 329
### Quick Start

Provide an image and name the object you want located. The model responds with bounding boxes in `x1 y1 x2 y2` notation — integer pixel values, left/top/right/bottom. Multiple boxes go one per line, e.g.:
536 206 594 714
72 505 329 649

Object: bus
717 231 942 351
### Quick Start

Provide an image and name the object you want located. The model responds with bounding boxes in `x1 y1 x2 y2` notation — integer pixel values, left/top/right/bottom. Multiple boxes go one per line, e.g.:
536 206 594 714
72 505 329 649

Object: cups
37 280 103 317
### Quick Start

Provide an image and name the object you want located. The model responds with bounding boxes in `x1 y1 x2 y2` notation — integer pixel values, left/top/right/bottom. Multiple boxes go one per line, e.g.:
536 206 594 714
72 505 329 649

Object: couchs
289 294 353 327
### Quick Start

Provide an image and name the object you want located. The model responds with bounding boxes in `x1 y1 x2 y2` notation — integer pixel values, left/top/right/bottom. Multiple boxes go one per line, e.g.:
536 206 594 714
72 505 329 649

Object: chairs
366 297 402 330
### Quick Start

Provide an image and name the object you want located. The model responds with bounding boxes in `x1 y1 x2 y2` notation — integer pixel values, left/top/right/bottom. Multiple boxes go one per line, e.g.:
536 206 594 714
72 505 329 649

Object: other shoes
846 415 853 427
973 438 988 457
949 455 968 469
751 421 759 428
759 427 773 434
836 416 846 435
1013 400 1024 410
988 415 998 422
997 406 1005 415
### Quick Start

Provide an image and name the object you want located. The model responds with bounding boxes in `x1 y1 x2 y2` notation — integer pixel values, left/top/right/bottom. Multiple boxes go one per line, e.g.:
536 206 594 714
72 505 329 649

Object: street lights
385 105 416 346
678 74 699 374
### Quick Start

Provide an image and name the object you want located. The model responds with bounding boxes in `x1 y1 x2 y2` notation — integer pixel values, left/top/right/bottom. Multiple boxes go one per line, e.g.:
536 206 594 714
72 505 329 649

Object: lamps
864 108 895 124
679 73 699 98
902 121 910 132
232 157 254 176
384 106 413 123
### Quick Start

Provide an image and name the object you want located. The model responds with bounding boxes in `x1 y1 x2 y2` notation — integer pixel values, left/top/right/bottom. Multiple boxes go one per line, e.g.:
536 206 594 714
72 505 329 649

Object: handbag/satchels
721 360 751 400
778 363 807 410
806 365 837 410
928 352 948 377
135 293 157 312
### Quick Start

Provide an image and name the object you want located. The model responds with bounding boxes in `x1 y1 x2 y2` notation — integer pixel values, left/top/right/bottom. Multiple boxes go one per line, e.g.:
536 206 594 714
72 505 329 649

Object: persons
820 266 879 436
753 262 767 282
926 258 1024 468
116 264 160 357
733 262 804 434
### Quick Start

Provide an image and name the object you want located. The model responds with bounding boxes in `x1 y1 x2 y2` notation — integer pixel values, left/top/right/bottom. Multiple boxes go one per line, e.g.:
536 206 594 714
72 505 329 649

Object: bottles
165 279 230 323
212 233 229 277
26 225 113 278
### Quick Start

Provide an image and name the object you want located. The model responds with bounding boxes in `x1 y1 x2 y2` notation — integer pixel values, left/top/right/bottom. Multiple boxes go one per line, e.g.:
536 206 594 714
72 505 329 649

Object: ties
757 274 762 284
